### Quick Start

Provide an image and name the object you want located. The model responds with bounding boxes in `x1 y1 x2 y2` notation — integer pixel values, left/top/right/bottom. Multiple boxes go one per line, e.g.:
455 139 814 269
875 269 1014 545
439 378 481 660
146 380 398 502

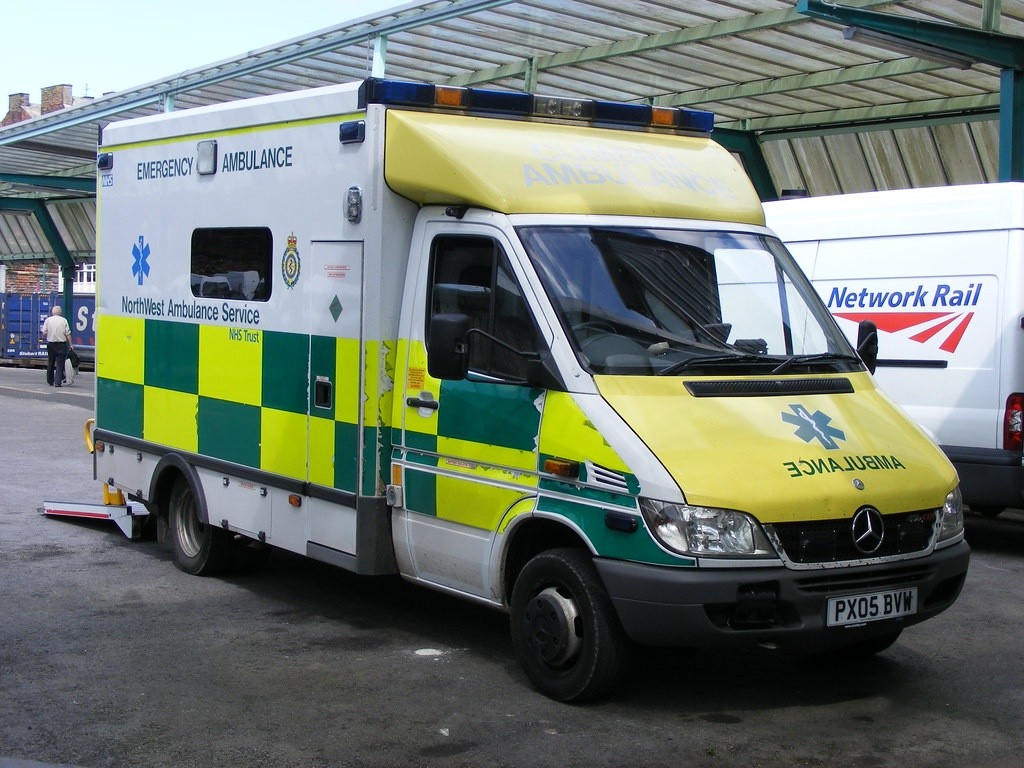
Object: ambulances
36 76 972 703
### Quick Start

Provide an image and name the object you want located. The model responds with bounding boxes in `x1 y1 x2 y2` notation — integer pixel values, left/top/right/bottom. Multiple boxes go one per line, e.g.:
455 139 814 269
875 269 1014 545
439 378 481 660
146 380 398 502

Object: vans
715 181 1024 515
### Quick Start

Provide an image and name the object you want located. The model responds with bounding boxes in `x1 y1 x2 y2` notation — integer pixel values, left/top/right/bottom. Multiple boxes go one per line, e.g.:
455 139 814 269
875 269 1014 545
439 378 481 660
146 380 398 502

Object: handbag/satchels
64 358 75 386
67 347 80 367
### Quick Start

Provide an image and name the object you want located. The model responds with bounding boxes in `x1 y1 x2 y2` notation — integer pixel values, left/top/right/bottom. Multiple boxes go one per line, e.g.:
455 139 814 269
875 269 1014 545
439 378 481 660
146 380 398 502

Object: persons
43 306 74 387
